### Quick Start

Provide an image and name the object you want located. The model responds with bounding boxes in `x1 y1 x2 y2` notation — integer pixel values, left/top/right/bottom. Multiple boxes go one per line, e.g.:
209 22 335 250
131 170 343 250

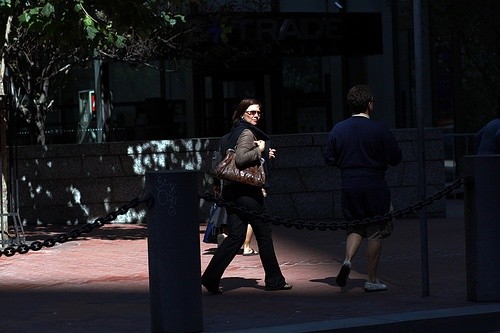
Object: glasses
370 97 375 103
245 110 263 116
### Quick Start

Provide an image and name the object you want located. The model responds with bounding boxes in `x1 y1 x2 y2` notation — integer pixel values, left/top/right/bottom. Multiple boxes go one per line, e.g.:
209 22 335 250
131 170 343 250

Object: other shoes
364 281 389 292
336 260 352 287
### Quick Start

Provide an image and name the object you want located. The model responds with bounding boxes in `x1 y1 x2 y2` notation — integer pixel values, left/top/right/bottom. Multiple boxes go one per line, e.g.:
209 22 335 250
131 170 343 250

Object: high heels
264 280 294 291
200 277 223 296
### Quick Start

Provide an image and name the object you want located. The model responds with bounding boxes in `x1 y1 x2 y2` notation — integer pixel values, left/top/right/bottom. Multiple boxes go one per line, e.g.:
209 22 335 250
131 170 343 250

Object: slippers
216 234 226 251
243 248 259 256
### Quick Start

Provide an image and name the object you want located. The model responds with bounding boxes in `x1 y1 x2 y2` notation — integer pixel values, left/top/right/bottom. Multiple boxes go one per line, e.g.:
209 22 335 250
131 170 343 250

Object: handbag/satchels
203 202 221 245
215 132 267 186
209 205 230 228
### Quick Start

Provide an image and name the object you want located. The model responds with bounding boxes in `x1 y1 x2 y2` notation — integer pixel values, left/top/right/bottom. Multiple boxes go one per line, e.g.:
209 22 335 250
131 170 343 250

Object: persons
211 131 261 258
322 83 405 291
200 98 294 292
